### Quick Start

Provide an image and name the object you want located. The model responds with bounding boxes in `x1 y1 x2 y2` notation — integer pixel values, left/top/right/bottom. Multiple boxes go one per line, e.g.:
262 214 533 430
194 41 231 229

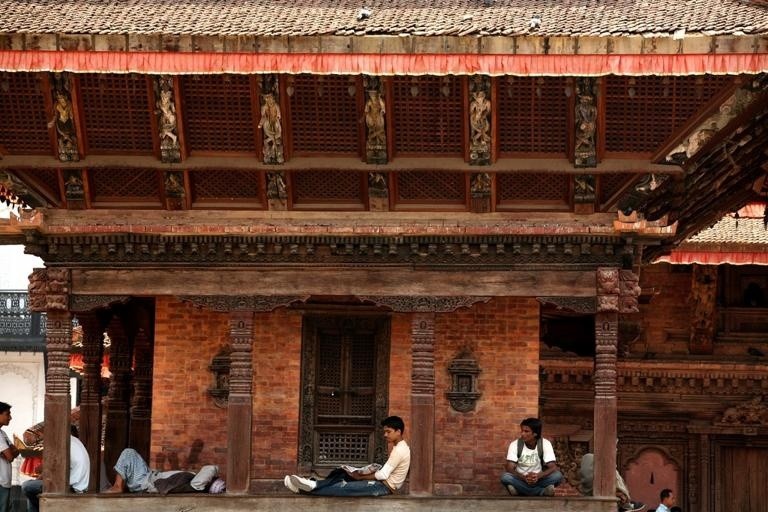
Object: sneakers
291 474 317 492
284 475 299 493
622 500 646 512
543 484 555 497
507 484 517 496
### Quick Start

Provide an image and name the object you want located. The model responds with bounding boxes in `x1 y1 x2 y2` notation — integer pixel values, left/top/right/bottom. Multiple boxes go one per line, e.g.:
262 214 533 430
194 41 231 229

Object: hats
209 478 225 493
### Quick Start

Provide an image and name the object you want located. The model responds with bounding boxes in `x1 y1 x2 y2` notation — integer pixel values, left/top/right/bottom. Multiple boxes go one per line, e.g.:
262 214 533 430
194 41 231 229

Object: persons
653 488 677 511
500 415 562 496
468 83 492 160
254 84 286 168
0 402 19 512
102 445 227 494
284 415 412 496
356 84 387 162
151 75 181 162
572 87 598 164
581 437 647 510
21 433 92 512
45 86 82 163
